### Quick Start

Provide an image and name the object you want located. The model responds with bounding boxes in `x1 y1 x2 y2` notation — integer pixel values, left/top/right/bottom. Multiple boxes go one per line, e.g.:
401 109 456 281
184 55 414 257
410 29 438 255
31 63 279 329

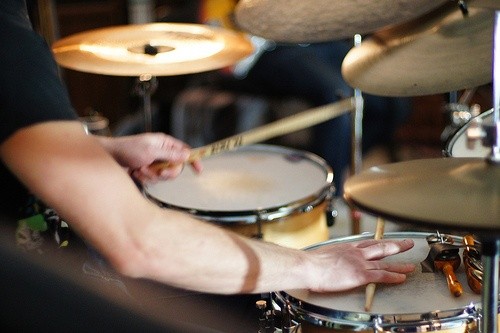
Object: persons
0 0 415 294
199 0 411 195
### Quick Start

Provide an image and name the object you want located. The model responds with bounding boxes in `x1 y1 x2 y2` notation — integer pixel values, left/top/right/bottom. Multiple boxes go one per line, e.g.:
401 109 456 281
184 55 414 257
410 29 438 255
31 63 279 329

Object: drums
270 231 497 333
139 143 337 250
445 106 500 162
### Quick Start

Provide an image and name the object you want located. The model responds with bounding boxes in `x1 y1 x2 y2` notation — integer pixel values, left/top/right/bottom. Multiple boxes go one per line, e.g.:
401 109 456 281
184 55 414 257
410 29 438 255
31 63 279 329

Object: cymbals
341 0 500 99
233 0 454 45
343 155 500 240
50 21 256 77
